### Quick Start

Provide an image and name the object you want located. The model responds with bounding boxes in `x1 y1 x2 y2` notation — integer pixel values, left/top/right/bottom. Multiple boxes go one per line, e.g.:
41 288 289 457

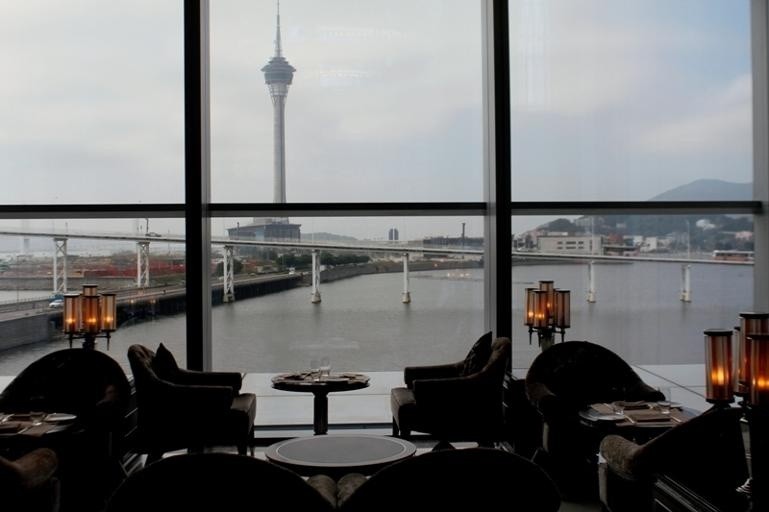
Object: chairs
108 448 339 509
390 332 513 437
3 350 131 446
526 340 668 502
128 342 257 462
598 407 749 508
338 445 562 510
3 448 66 509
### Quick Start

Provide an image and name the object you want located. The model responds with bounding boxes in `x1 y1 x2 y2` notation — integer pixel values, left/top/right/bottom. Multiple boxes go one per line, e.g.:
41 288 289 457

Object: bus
603 246 640 257
713 250 754 261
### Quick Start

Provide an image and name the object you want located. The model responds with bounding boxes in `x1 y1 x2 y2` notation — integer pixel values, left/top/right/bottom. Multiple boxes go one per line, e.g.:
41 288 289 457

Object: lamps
523 281 571 354
705 312 769 496
61 284 118 352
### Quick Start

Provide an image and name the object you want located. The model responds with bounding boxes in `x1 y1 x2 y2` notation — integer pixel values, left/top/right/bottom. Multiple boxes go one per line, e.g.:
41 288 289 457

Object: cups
310 357 330 381
612 387 626 416
657 386 671 416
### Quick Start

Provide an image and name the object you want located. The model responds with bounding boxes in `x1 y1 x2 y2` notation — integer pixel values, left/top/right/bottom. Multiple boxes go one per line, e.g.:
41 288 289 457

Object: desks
4 411 79 447
573 400 690 512
266 436 417 479
271 372 369 436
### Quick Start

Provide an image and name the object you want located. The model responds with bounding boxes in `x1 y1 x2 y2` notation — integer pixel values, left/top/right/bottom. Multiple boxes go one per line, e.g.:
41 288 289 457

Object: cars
49 299 64 308
